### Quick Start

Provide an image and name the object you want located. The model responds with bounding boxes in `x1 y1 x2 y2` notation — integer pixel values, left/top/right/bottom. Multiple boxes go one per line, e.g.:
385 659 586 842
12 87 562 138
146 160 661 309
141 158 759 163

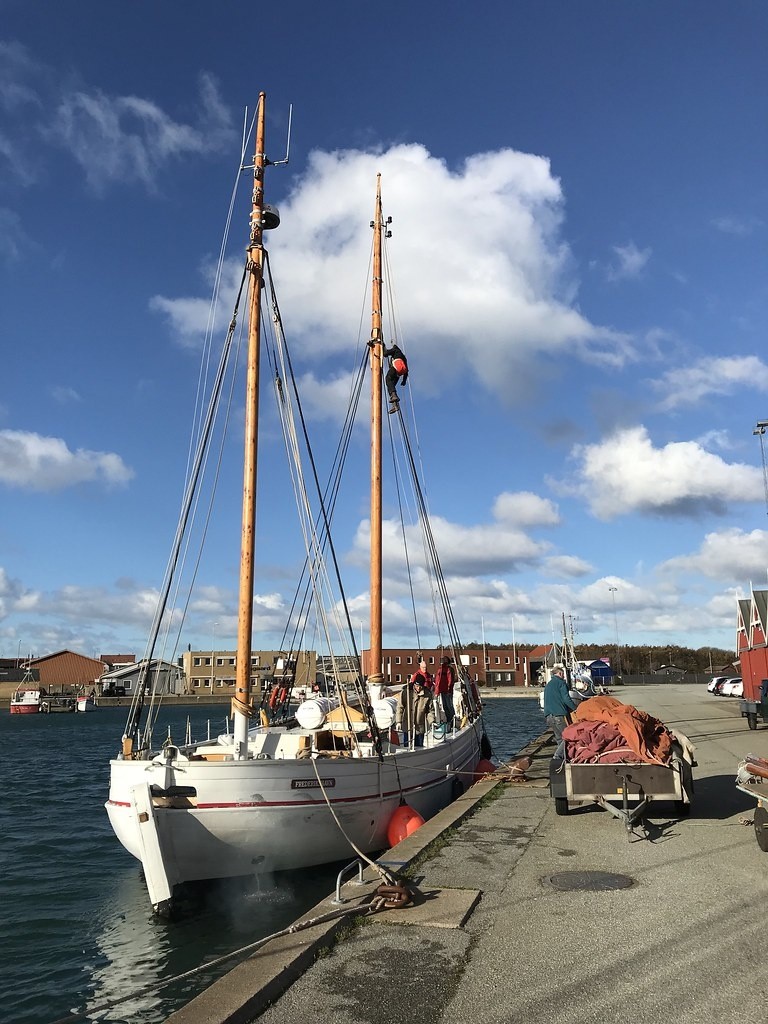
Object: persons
544 667 576 747
434 656 457 728
396 674 435 747
411 661 433 692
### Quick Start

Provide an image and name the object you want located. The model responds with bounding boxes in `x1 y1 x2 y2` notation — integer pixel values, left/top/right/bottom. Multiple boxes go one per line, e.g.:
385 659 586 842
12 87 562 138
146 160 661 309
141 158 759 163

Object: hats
414 674 424 685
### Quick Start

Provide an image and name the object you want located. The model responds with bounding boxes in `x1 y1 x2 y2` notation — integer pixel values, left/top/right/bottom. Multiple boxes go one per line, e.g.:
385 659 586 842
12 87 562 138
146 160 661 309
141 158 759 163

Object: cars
708 676 744 699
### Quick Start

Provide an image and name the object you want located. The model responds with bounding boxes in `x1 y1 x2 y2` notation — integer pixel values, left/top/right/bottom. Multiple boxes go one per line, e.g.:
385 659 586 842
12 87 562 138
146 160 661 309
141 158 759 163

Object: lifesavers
269 685 286 713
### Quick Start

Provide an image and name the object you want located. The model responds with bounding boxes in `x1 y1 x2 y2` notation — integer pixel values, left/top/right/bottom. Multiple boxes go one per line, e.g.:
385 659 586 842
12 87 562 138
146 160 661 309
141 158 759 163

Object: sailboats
105 90 492 915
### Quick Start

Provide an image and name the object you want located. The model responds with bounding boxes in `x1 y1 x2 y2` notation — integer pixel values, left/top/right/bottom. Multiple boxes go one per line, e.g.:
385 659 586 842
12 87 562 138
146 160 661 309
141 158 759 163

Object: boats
538 612 597 709
10 650 97 714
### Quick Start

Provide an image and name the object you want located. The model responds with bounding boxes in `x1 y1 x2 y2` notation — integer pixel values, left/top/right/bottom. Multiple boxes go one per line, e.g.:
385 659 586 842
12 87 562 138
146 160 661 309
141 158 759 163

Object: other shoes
553 754 561 761
